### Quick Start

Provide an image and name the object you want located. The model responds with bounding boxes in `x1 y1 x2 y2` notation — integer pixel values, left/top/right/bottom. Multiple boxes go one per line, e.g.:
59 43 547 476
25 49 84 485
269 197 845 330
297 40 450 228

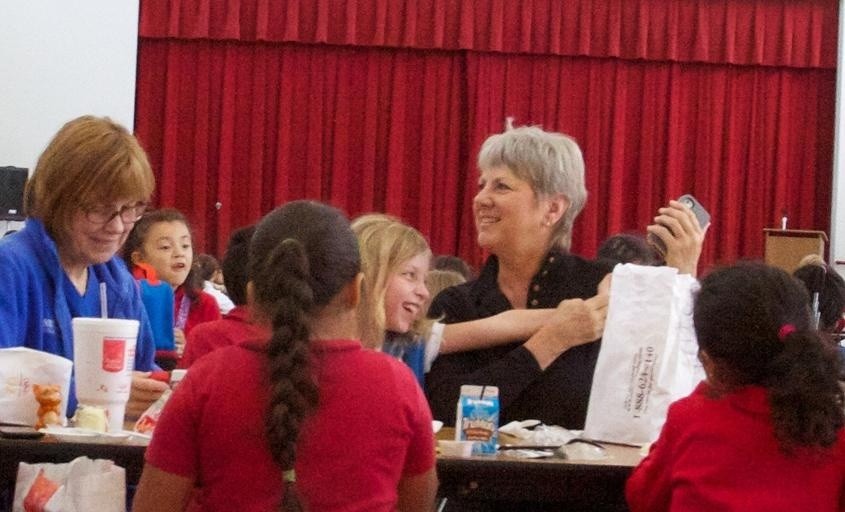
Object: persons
422 125 712 512
122 205 258 372
0 114 169 421
418 254 473 320
350 214 560 377
623 260 844 511
793 260 845 334
131 198 439 511
596 234 655 267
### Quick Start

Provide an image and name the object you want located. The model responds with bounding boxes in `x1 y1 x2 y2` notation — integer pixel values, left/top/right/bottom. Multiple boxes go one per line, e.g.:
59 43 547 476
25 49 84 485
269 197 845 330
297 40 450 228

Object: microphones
781 215 788 230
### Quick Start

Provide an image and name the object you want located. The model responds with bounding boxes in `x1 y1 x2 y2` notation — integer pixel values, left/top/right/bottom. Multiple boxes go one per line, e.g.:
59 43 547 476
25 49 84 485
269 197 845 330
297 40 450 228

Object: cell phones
647 193 712 258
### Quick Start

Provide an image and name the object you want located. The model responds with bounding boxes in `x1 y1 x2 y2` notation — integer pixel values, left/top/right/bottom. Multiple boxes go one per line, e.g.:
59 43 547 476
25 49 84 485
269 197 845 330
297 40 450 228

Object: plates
37 427 150 446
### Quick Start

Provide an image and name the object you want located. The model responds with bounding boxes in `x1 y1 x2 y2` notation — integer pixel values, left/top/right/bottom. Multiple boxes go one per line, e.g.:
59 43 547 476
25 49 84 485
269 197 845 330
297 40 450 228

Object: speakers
0 165 28 221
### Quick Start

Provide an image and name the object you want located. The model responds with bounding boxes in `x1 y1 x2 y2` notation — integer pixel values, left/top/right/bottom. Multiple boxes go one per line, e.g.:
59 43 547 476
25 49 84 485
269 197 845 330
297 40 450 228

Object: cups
73 315 139 435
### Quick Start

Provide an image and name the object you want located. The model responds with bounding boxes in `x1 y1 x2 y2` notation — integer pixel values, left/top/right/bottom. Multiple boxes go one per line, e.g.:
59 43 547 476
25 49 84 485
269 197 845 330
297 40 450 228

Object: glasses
72 195 147 224
